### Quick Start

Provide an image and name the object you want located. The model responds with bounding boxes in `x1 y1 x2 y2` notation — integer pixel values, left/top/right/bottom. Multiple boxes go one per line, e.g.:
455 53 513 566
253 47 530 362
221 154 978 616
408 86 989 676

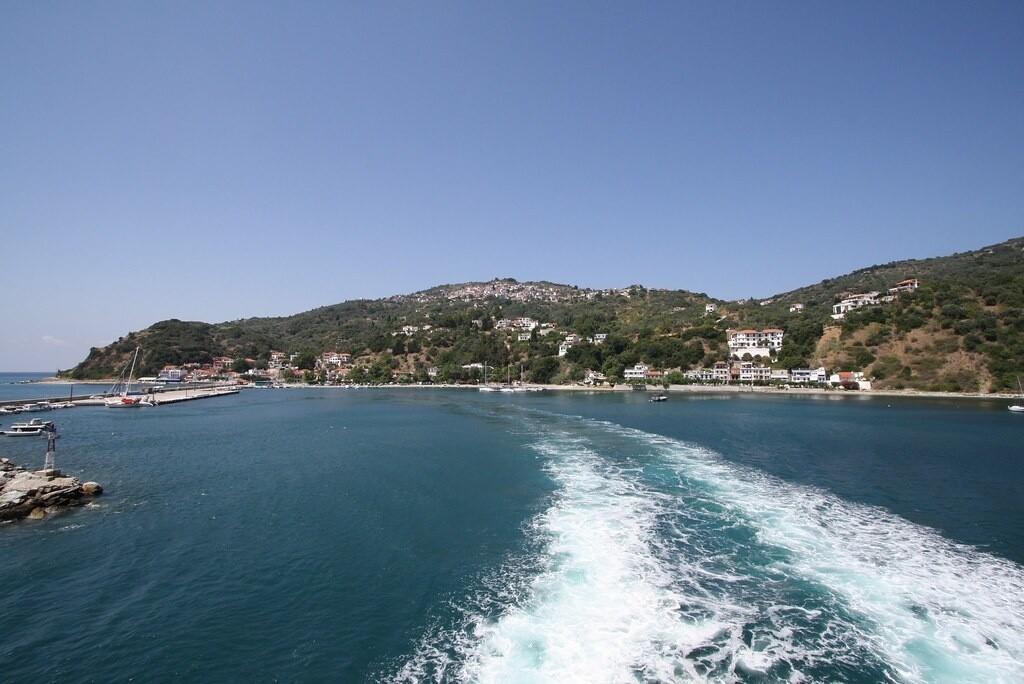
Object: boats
104 346 155 408
0 401 76 415
651 392 669 402
4 417 57 437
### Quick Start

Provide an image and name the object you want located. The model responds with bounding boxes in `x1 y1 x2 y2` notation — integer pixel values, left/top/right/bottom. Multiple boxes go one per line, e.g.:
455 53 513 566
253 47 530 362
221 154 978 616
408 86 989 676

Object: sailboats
1007 376 1024 412
